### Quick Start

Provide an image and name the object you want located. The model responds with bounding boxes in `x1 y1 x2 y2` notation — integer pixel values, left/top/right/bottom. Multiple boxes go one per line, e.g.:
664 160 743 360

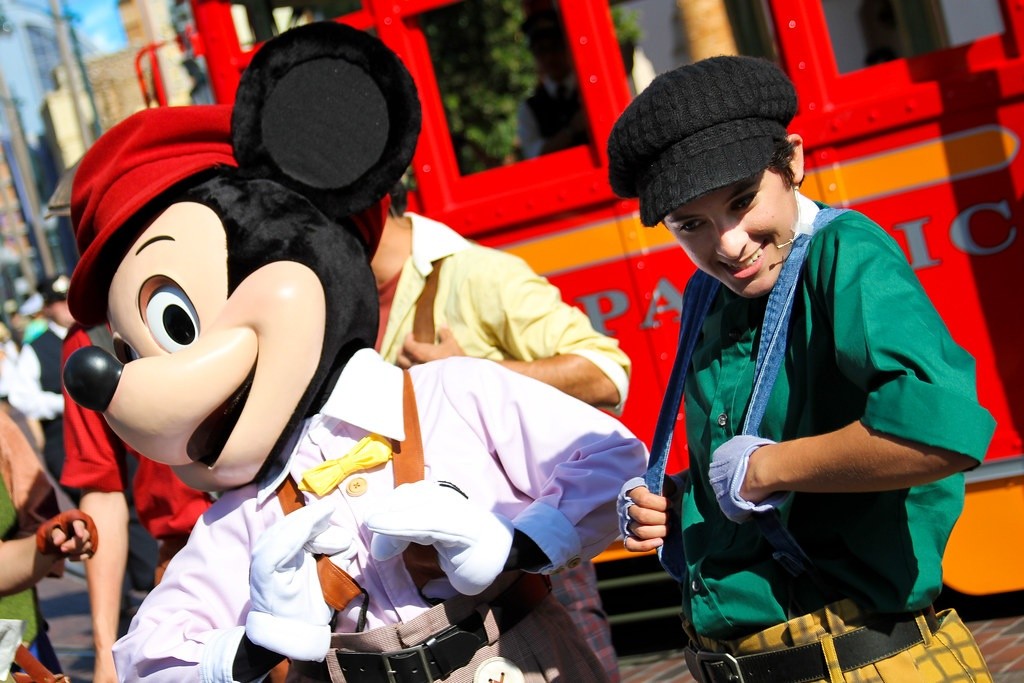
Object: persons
607 56 997 683
372 180 633 683
0 272 291 683
515 0 659 159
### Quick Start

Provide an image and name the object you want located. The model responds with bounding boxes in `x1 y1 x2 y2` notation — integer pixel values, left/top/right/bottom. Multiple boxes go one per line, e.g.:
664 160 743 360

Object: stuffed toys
65 21 648 683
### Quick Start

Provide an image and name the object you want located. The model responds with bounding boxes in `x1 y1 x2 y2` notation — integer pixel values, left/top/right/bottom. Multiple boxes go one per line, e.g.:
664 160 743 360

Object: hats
606 54 796 227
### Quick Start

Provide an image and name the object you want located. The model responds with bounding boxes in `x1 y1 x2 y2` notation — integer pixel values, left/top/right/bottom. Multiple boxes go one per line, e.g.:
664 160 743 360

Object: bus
129 1 1024 646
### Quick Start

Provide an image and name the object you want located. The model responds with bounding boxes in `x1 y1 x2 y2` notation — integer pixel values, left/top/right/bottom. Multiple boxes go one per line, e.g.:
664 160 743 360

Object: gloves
709 435 793 524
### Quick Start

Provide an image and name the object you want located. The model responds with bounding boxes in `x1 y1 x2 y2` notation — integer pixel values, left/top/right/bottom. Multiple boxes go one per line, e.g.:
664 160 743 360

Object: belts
682 603 940 683
